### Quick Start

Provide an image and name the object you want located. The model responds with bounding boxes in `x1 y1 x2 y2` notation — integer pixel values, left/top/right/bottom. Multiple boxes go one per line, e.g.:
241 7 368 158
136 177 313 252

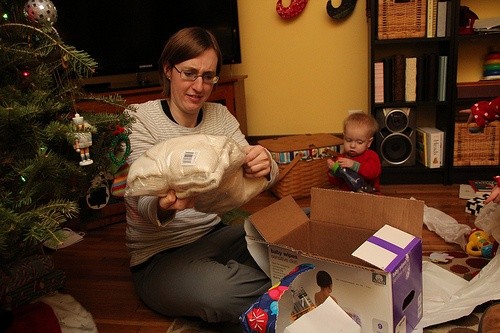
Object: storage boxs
250 186 432 333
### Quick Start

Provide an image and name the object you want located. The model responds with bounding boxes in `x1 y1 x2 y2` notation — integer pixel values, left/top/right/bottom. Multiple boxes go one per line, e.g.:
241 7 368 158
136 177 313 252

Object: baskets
256 134 345 201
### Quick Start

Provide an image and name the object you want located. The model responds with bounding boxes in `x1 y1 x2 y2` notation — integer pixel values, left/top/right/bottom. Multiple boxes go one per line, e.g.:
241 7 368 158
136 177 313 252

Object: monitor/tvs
49 0 241 94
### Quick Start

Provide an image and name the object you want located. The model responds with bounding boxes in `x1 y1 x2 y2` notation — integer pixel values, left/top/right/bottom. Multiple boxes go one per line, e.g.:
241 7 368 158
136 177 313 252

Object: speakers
373 107 417 167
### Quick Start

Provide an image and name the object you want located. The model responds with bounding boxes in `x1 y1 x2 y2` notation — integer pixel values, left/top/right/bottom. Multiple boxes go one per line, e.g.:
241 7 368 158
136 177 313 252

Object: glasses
173 65 218 83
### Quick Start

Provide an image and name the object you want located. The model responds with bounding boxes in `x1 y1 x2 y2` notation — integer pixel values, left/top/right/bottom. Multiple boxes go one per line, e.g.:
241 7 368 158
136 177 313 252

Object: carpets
167 248 494 333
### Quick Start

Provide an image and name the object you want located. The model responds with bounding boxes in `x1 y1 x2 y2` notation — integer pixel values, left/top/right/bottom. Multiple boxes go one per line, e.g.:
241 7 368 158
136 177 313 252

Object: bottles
329 162 380 196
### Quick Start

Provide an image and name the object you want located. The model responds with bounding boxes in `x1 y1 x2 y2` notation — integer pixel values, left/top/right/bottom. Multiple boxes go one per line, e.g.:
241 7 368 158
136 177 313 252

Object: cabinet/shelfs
72 73 247 197
370 0 500 184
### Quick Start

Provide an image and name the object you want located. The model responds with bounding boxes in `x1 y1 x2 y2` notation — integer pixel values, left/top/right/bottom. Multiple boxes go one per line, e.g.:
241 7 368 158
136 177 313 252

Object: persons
315 271 338 308
73 114 93 166
326 113 383 196
124 27 279 324
